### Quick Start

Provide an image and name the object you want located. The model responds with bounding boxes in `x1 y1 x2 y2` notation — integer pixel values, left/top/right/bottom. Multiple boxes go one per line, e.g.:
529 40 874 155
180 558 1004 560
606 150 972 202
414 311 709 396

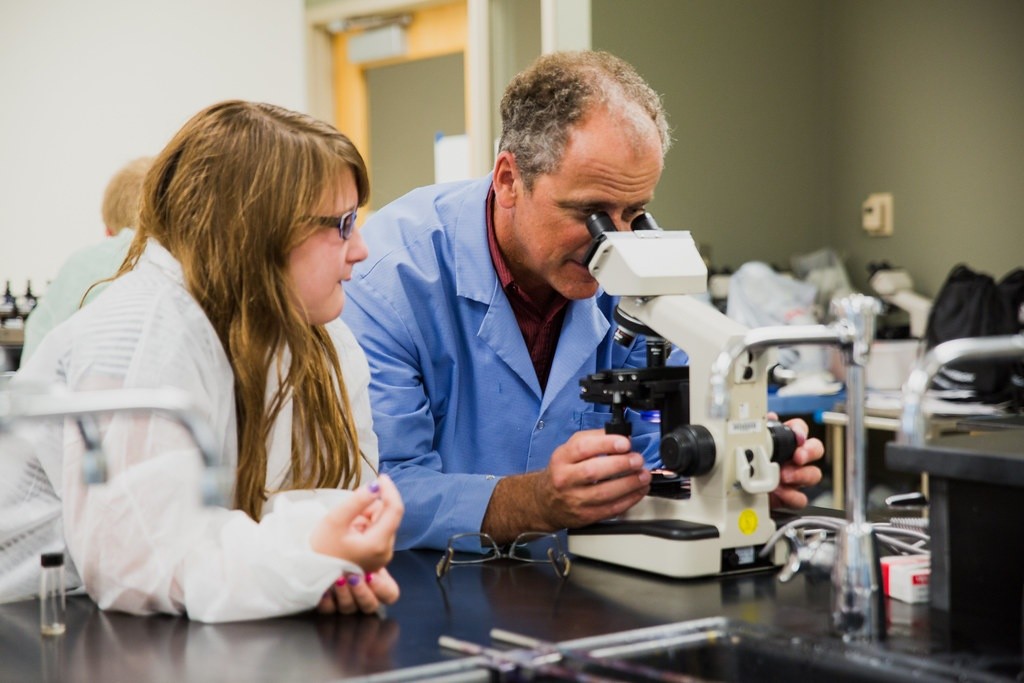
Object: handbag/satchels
921 265 1024 415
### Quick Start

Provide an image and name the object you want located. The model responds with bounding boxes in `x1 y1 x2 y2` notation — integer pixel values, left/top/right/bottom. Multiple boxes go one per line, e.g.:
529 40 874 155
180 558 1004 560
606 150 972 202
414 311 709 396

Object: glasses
436 533 571 579
298 211 359 240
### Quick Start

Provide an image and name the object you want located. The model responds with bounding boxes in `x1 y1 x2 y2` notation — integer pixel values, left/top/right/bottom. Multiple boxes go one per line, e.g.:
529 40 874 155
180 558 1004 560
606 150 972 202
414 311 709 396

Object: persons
20 155 155 372
0 97 405 626
339 49 825 552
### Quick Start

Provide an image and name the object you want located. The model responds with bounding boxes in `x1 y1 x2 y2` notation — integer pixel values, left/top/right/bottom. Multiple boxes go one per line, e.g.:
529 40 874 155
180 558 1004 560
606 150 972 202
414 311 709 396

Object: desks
0 549 927 683
